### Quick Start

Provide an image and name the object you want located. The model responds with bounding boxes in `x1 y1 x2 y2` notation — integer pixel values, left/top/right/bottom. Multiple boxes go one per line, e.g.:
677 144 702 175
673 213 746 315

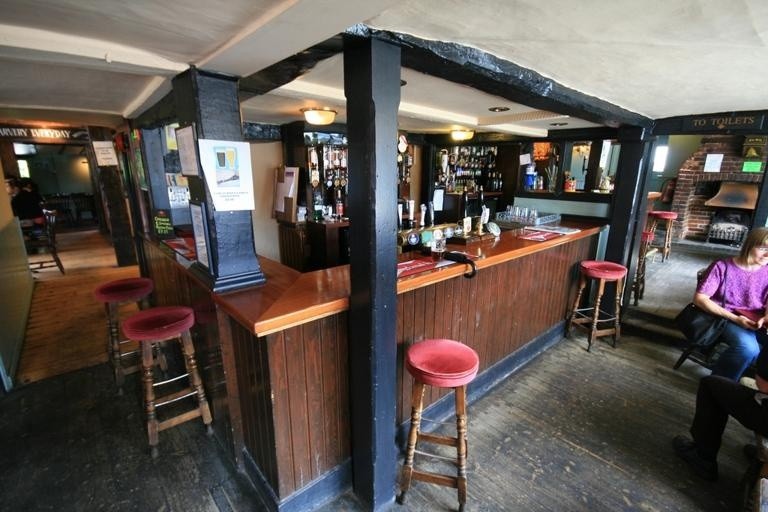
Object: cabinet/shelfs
284 144 410 266
422 145 503 223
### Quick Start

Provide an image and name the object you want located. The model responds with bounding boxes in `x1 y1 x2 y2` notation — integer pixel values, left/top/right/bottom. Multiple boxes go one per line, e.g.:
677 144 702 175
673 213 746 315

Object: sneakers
743 444 764 468
672 435 718 482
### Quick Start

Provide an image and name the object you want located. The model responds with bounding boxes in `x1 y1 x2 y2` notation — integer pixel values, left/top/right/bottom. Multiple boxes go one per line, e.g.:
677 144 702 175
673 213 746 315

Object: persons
4 176 44 254
694 225 768 382
676 341 768 481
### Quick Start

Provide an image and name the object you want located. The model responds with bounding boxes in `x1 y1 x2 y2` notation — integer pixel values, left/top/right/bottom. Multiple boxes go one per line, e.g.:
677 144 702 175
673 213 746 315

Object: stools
632 232 654 306
96 277 169 397
647 210 678 263
123 306 214 459
400 339 479 512
566 261 628 352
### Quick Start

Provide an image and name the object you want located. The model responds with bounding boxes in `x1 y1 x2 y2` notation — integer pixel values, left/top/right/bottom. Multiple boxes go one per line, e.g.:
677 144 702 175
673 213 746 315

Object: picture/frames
175 122 202 179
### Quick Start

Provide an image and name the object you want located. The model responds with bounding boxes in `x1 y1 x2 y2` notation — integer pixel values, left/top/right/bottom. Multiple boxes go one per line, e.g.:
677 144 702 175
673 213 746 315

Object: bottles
474 184 486 232
341 152 347 177
498 173 502 192
325 151 334 181
335 190 344 220
487 172 492 192
459 185 472 234
334 148 340 179
492 172 496 192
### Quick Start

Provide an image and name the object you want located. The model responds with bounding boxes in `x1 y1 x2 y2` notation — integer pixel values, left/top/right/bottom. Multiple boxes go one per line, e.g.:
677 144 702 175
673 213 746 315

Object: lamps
451 130 474 141
299 107 338 125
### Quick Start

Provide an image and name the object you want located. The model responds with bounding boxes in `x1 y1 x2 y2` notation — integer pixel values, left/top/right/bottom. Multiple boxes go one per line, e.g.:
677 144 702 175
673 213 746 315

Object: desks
648 192 661 198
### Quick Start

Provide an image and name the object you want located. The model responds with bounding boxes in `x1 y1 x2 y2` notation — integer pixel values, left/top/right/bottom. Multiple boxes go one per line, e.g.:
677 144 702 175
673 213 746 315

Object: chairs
673 268 763 379
10 192 99 277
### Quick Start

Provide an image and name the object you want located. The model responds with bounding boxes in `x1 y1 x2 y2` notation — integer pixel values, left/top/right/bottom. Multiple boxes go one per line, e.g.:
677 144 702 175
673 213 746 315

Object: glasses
754 245 768 256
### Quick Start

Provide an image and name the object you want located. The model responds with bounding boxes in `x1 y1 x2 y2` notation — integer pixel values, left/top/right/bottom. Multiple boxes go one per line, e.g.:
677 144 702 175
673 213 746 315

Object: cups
432 238 446 262
529 208 538 225
513 206 520 220
521 207 529 222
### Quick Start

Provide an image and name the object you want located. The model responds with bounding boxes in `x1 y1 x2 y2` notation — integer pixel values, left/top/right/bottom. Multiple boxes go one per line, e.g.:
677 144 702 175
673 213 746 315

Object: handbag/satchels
673 302 728 355
